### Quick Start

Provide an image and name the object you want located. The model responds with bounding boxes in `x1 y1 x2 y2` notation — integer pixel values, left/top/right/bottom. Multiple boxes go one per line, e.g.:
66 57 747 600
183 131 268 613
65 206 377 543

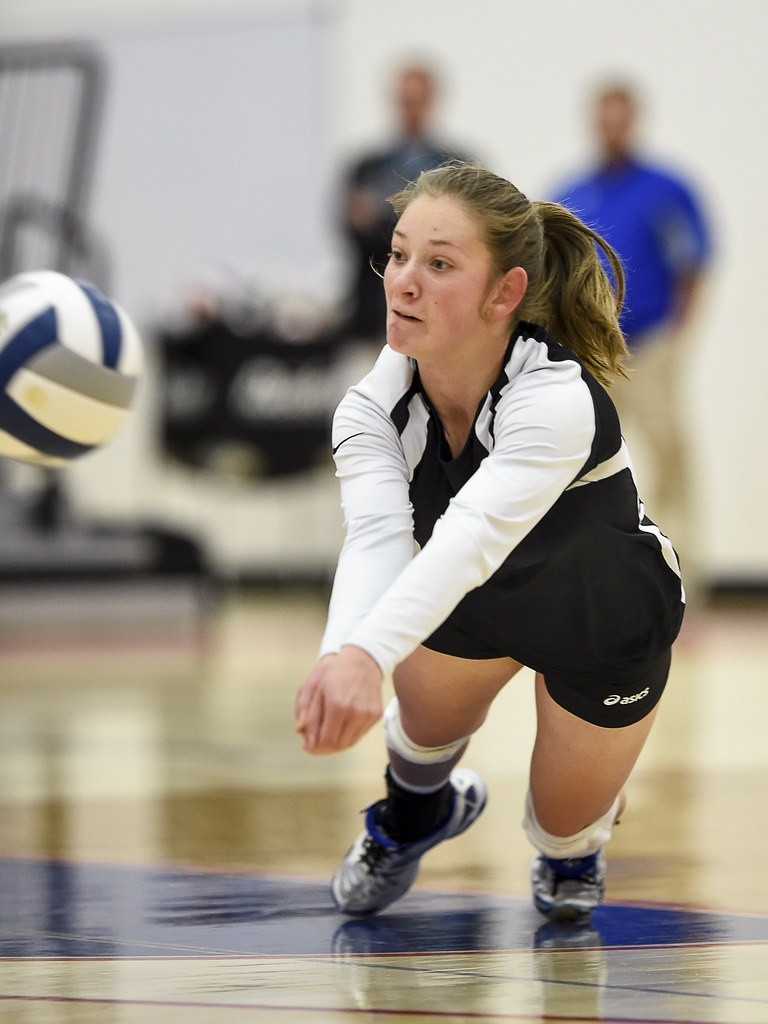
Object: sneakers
537 925 603 1020
332 921 417 1012
531 853 605 922
329 768 487 916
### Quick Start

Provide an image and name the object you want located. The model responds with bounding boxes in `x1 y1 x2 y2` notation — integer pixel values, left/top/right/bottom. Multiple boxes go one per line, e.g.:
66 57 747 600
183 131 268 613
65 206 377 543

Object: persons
292 166 686 926
338 68 478 363
542 77 720 617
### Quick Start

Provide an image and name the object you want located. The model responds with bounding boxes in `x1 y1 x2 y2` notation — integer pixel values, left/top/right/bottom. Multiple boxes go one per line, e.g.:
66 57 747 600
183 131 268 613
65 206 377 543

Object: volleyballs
0 269 143 468
